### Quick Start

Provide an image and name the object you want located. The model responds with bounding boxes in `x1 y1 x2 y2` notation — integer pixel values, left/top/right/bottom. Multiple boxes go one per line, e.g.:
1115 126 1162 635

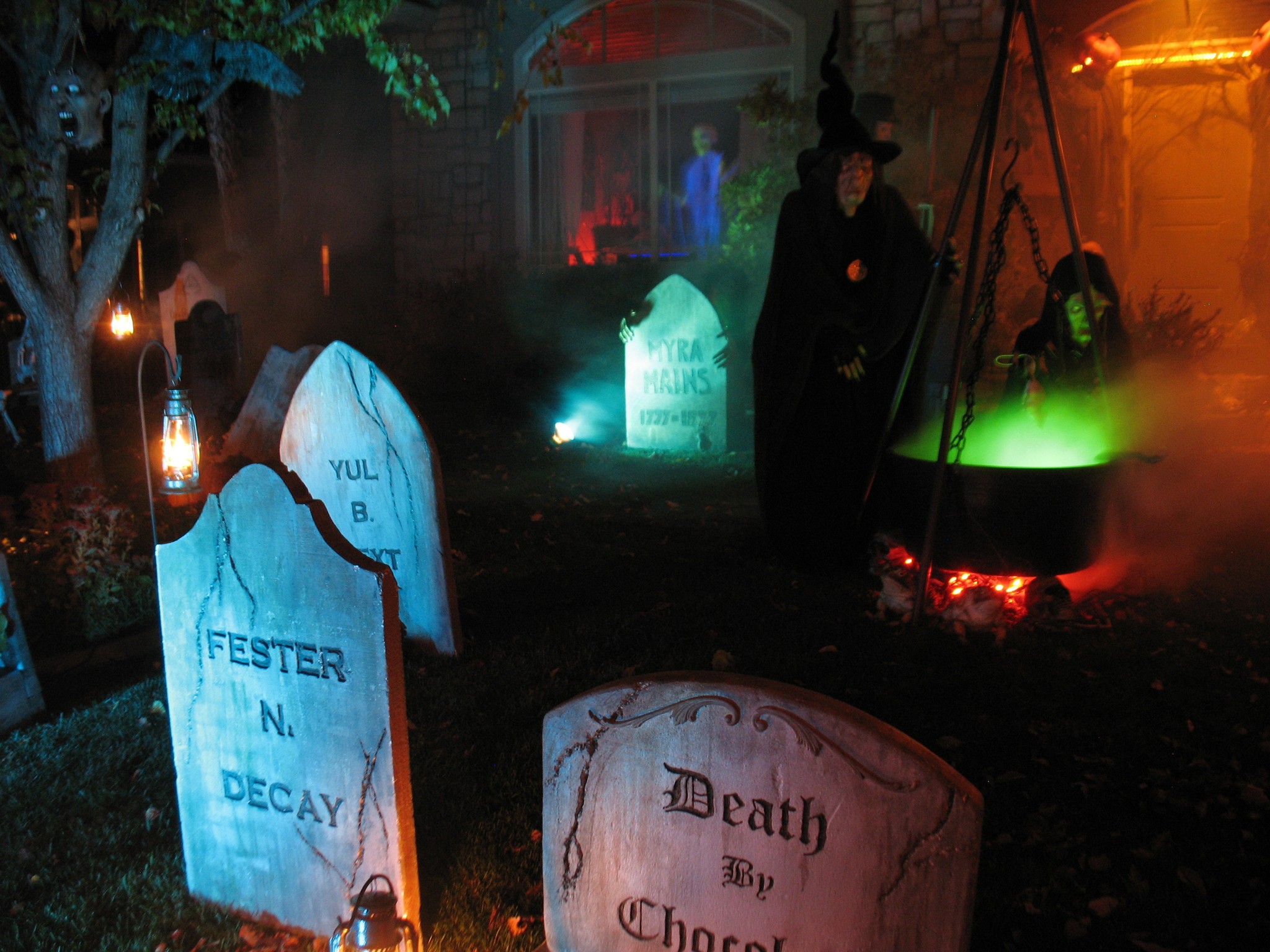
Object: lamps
156 380 203 493
110 291 134 341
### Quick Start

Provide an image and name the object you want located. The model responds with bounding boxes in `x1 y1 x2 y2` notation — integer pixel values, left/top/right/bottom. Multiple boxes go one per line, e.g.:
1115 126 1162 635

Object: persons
47 60 111 148
856 93 901 141
679 125 725 247
1004 251 1131 404
753 135 963 568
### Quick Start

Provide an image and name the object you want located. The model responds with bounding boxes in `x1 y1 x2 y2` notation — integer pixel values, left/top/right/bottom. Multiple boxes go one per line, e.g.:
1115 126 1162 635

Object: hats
852 93 904 125
795 121 902 189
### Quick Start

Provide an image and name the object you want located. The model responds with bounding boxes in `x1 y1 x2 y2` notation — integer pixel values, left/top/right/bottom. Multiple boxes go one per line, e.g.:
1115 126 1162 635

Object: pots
887 417 1122 582
